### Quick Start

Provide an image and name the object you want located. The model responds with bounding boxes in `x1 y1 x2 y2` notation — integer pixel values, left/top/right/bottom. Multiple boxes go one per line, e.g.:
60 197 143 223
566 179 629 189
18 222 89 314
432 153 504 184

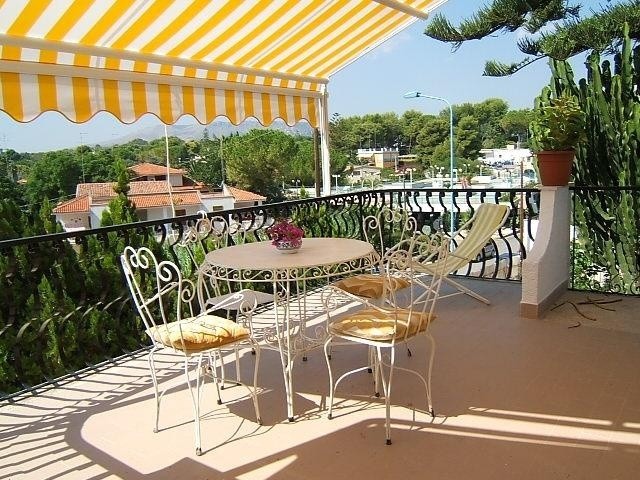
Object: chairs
172 216 286 389
323 233 451 445
384 203 510 310
120 246 263 455
321 202 417 374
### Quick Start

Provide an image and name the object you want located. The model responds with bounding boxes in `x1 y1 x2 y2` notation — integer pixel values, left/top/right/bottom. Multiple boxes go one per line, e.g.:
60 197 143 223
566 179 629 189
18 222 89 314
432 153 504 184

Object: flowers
267 221 303 246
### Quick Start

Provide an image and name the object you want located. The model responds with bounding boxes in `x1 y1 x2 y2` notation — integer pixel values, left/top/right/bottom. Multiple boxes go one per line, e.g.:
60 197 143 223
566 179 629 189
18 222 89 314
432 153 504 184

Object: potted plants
521 86 588 186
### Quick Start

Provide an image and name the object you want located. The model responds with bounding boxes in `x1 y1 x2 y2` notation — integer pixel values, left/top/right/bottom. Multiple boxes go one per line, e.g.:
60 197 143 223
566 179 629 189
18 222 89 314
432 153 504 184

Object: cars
455 238 497 263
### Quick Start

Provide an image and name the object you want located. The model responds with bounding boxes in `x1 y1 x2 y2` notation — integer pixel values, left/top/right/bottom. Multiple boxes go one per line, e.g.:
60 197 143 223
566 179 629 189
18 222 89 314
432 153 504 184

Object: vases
274 241 301 253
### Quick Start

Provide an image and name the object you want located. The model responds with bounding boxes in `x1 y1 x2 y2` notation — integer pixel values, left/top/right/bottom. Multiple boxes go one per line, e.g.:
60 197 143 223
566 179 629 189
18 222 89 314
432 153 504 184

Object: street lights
397 87 454 252
332 175 341 193
292 179 300 195
405 163 494 186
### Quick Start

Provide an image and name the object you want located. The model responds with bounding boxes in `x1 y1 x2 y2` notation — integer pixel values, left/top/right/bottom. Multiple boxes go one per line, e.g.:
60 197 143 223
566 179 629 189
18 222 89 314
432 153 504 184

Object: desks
195 237 382 420
